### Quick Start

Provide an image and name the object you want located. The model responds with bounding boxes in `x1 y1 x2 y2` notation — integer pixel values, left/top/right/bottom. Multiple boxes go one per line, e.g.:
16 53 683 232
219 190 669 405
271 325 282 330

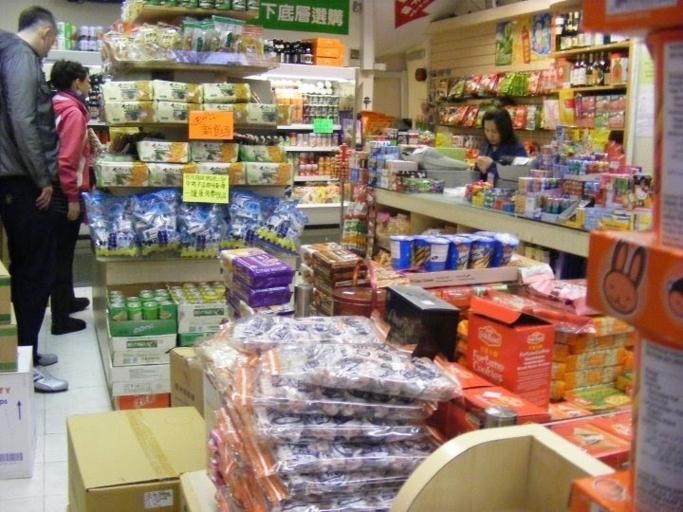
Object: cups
389 230 522 274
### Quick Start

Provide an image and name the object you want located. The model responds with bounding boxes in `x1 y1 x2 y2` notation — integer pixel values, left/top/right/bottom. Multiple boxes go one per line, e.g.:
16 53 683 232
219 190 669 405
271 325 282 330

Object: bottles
554 11 610 87
531 14 550 54
519 25 532 64
270 41 313 65
295 283 314 319
611 59 623 83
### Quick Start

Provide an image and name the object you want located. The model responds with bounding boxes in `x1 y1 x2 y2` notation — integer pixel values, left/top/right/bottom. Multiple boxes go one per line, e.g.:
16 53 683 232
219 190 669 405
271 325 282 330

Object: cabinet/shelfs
548 1 655 169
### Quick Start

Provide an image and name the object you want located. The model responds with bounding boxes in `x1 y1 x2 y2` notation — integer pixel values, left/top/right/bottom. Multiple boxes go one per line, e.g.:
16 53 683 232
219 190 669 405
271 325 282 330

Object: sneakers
32 366 67 393
70 296 89 313
51 316 86 335
37 352 57 367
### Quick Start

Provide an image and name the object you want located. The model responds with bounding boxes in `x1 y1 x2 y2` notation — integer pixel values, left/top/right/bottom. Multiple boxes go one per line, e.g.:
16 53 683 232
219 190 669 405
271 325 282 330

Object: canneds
109 281 227 321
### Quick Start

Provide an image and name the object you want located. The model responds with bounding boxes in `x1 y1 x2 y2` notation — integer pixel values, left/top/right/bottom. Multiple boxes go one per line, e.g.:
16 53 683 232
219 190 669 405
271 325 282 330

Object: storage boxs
66 406 210 512
1 259 38 480
164 345 205 415
179 466 218 511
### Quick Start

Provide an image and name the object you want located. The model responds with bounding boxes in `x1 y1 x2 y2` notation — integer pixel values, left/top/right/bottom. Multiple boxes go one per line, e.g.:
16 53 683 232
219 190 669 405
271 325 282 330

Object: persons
0 6 68 393
46 59 97 335
81 103 102 188
471 107 527 180
